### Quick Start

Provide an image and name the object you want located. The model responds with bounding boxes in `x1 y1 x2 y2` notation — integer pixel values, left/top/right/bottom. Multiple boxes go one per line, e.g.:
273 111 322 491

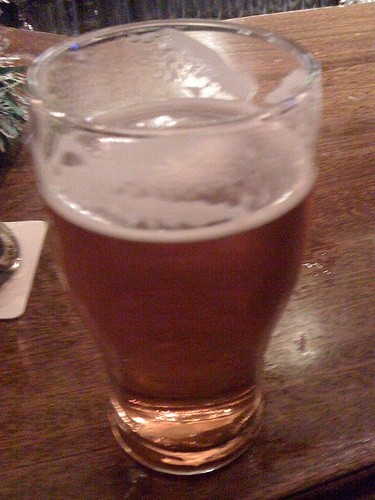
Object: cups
25 19 322 476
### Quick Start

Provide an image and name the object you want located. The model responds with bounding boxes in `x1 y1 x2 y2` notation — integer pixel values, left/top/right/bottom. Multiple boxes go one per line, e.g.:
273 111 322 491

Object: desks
0 1 375 500
